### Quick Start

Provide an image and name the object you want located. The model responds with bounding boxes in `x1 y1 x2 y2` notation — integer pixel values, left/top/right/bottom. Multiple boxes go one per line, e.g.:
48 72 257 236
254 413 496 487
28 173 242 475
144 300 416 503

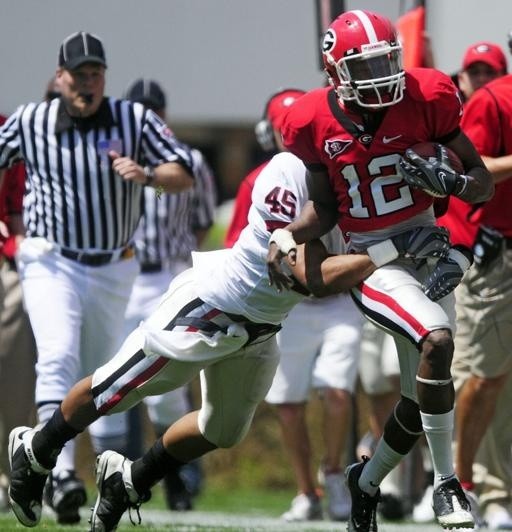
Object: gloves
389 225 450 260
399 144 467 199
422 243 473 301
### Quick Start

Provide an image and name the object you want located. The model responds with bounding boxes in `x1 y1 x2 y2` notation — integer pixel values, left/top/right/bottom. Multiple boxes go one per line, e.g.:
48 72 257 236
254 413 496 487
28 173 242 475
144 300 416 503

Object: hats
131 82 165 109
59 33 106 73
463 42 507 73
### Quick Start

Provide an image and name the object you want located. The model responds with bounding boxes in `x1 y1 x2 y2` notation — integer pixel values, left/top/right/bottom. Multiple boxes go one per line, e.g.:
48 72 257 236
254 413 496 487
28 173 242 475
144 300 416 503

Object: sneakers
166 463 190 509
282 435 512 532
6 426 151 532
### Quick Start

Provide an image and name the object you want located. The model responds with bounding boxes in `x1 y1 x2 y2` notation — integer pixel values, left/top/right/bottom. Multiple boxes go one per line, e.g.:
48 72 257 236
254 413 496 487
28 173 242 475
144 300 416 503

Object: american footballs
403 142 465 174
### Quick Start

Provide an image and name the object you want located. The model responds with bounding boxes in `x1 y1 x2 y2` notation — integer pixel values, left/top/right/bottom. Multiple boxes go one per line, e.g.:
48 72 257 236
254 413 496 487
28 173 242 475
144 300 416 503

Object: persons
125 78 216 512
8 147 454 531
357 38 511 532
0 29 200 528
258 7 494 531
0 76 66 514
226 90 360 522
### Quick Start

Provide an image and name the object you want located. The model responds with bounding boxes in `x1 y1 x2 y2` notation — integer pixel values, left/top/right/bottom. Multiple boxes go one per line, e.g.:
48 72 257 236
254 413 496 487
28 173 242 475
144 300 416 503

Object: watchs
141 166 154 186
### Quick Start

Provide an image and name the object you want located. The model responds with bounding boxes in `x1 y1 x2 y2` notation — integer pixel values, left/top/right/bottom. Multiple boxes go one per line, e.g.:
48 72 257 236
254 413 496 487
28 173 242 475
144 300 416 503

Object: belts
62 248 133 264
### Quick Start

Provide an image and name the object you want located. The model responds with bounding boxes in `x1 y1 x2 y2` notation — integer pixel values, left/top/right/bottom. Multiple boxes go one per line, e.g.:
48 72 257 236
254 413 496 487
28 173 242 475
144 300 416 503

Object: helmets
321 10 406 108
264 90 307 121
